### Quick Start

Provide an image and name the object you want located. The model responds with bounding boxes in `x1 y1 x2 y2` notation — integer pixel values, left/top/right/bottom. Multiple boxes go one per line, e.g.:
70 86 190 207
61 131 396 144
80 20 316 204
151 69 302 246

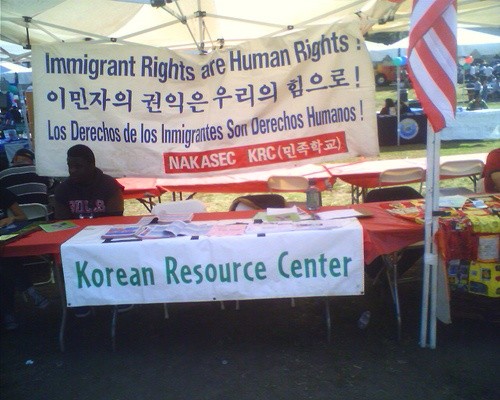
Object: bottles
306 180 320 210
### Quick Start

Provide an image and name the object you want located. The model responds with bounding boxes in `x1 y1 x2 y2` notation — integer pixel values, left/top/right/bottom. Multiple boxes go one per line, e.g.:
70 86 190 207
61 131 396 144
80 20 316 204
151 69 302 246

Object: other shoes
32 294 49 309
4 314 17 331
75 307 89 316
118 304 131 313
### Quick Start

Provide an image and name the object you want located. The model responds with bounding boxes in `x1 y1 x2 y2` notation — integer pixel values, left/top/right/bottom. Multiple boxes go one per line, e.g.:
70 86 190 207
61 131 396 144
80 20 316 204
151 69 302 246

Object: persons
54 144 134 316
0 148 58 329
485 148 500 195
382 61 500 115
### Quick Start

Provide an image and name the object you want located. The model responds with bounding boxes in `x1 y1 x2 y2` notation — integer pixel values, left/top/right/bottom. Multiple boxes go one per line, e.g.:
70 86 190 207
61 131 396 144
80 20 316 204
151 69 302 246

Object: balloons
393 55 407 66
459 55 474 70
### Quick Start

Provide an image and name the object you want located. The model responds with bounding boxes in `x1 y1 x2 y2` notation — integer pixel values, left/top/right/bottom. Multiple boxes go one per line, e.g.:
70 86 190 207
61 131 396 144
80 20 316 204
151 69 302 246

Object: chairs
268 176 309 208
367 186 427 305
378 167 424 195
439 160 484 197
151 199 224 319
228 194 295 309
6 203 55 302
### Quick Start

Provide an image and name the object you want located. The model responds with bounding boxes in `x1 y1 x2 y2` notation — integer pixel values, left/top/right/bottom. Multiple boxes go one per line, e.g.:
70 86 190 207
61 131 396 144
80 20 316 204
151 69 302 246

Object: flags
406 0 459 133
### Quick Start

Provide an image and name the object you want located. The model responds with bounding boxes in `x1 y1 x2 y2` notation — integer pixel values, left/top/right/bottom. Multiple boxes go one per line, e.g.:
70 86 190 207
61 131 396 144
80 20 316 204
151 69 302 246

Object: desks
0 109 500 351
